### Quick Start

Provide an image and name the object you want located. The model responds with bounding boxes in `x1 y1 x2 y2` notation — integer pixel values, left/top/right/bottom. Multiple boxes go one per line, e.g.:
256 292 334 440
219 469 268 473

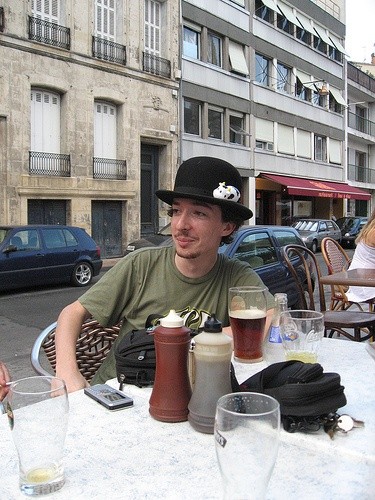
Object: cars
290 219 341 255
333 216 369 248
159 224 315 318
124 220 171 255
0 225 103 295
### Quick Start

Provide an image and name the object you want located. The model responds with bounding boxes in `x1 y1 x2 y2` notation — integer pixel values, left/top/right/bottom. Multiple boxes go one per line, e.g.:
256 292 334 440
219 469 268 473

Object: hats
155 156 254 222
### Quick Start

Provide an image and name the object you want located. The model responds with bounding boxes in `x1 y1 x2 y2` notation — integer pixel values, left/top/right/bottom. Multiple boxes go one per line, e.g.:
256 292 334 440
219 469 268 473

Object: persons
0 360 11 402
50 156 298 401
344 205 375 303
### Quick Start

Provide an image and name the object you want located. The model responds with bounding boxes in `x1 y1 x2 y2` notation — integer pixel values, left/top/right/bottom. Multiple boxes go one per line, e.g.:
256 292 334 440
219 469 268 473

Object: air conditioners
256 142 274 152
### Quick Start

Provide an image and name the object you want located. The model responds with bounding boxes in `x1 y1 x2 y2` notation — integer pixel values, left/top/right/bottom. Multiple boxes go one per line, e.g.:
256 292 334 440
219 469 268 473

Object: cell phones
84 384 133 410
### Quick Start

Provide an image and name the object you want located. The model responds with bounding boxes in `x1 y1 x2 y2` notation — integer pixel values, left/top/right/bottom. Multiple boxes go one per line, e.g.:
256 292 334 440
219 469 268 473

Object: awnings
329 88 347 105
261 0 351 58
260 173 371 200
297 71 318 92
229 40 249 76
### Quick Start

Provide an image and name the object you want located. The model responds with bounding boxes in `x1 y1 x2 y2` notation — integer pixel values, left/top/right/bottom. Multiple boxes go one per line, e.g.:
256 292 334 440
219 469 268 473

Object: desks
319 268 375 288
0 335 375 500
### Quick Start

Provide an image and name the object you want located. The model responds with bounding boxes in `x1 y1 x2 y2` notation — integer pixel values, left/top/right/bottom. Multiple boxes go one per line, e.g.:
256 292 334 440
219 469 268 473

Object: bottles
148 309 194 423
264 292 298 367
187 313 236 433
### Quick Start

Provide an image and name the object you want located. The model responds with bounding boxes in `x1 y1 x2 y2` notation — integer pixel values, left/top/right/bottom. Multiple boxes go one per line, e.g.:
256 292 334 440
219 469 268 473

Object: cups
279 309 324 367
213 392 282 499
228 286 268 362
4 376 68 495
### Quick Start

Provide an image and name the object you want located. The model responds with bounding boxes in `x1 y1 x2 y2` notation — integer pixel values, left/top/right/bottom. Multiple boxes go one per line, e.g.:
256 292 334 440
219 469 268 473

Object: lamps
294 79 329 95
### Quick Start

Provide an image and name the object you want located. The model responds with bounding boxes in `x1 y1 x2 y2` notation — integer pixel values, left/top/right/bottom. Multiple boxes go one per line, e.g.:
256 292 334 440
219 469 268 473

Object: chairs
12 236 22 250
31 237 37 248
321 236 375 342
247 256 265 268
282 244 375 344
31 315 125 385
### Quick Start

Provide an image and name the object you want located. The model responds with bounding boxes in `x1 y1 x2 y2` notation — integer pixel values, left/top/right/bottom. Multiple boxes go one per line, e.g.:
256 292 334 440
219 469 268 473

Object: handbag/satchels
230 359 347 434
113 314 202 390
